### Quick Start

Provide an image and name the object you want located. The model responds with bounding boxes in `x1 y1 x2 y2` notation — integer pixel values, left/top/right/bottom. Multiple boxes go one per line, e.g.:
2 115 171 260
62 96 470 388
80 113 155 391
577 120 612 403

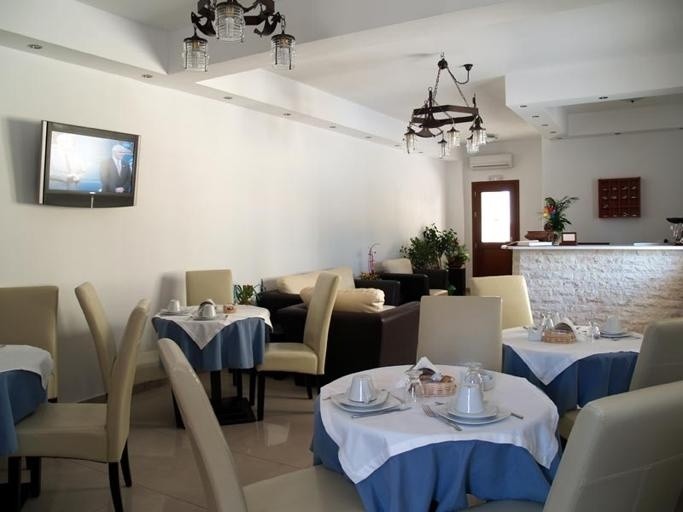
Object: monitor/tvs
36 120 141 210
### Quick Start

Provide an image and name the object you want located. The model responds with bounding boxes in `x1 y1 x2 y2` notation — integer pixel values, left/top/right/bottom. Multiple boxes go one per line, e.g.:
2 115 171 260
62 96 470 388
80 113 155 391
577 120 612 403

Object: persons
96 144 131 194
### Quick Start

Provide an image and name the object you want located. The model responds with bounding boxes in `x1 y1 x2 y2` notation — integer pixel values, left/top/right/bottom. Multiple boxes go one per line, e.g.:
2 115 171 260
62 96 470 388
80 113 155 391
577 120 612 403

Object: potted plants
443 244 469 299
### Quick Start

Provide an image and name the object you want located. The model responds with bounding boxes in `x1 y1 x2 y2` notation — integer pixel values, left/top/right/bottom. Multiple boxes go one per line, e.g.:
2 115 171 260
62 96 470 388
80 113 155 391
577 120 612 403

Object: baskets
223 304 237 312
411 373 457 397
543 326 576 343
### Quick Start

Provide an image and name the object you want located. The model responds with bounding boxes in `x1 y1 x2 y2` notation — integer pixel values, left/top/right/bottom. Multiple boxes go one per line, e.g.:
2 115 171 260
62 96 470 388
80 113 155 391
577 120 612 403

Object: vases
549 229 562 247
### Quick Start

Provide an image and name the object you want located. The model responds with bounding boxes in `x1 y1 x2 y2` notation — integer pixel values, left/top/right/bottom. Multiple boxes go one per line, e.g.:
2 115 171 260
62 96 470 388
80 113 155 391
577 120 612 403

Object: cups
402 369 427 409
531 310 545 329
454 383 488 415
462 361 485 395
346 373 377 404
585 320 600 344
166 299 181 312
606 317 620 331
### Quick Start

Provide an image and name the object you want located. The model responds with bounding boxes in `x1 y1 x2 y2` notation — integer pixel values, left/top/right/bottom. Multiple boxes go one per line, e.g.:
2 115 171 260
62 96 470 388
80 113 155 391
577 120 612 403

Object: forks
421 402 462 433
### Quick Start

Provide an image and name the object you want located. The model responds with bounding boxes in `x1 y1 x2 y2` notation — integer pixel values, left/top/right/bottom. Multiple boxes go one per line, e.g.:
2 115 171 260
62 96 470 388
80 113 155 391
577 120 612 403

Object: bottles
544 312 554 330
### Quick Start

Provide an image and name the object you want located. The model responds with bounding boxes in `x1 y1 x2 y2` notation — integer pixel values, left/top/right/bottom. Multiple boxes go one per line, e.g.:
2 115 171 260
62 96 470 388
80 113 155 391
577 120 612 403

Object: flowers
540 194 582 236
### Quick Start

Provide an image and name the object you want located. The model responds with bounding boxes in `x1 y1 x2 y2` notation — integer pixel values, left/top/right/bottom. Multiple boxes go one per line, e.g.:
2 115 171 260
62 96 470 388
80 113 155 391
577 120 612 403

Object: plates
436 401 513 426
448 403 499 418
601 328 628 335
598 326 633 338
335 390 388 406
329 388 400 413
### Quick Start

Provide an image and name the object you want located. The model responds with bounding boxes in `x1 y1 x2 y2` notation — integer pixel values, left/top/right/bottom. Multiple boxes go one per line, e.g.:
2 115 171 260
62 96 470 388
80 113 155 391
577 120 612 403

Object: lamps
178 0 297 74
396 55 491 160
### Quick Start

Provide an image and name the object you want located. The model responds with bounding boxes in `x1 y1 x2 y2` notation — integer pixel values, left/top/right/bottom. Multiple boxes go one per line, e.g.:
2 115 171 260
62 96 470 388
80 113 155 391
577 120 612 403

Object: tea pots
197 304 217 319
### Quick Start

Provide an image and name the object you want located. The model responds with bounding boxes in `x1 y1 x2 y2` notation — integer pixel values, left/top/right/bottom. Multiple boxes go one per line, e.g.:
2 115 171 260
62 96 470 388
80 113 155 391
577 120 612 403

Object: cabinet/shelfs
597 176 642 219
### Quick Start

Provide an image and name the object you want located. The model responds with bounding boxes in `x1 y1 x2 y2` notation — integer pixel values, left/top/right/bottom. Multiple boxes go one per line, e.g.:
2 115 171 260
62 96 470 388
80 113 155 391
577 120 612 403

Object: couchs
257 257 450 399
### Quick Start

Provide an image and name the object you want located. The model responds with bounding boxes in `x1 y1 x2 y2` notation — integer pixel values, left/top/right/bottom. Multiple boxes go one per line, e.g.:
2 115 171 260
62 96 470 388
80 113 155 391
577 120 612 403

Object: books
516 240 552 246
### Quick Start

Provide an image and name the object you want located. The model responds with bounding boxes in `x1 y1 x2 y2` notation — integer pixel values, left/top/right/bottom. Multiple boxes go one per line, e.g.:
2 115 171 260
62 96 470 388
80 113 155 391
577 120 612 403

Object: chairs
467 273 533 334
627 316 681 392
416 294 503 373
451 377 682 512
183 268 236 308
257 270 342 422
7 296 150 507
73 280 116 401
153 336 370 511
1 284 62 474
